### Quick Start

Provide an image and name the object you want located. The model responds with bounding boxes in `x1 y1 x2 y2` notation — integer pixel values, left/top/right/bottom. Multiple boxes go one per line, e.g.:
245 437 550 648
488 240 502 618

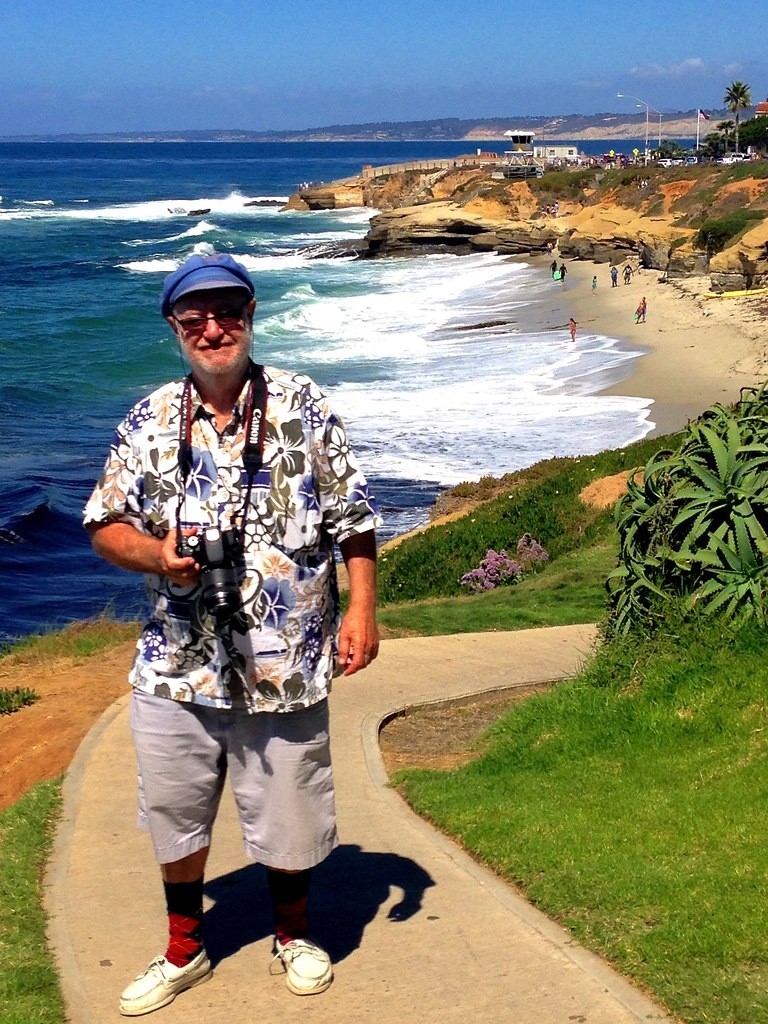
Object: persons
550 261 557 277
559 263 567 282
570 317 576 342
591 276 597 295
82 255 385 1015
635 297 646 324
622 264 634 285
547 240 553 255
611 267 618 287
541 202 559 218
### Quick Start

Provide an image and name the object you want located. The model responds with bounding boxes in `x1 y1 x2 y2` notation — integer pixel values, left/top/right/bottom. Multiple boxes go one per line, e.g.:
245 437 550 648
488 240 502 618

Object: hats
161 253 255 317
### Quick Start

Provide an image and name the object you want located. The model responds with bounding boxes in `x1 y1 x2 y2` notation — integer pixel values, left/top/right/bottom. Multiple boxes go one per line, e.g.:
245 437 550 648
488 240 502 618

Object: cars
657 156 700 169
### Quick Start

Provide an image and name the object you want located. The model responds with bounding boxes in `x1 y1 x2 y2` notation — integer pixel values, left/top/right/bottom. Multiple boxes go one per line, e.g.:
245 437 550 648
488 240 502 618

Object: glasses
170 302 249 334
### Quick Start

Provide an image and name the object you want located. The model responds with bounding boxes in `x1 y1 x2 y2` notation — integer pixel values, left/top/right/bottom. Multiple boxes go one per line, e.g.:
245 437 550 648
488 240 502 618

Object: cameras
174 526 247 617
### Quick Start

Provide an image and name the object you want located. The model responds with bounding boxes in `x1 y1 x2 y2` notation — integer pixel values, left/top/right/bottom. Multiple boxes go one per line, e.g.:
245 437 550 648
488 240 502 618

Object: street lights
636 104 648 168
617 95 664 158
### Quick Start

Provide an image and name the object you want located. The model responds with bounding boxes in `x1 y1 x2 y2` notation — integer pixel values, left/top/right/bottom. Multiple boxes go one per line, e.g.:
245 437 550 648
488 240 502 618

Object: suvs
716 152 752 165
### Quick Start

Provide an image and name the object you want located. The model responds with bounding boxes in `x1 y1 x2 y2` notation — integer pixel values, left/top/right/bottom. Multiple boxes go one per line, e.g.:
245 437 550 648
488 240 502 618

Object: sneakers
269 937 333 995
118 946 213 1015
